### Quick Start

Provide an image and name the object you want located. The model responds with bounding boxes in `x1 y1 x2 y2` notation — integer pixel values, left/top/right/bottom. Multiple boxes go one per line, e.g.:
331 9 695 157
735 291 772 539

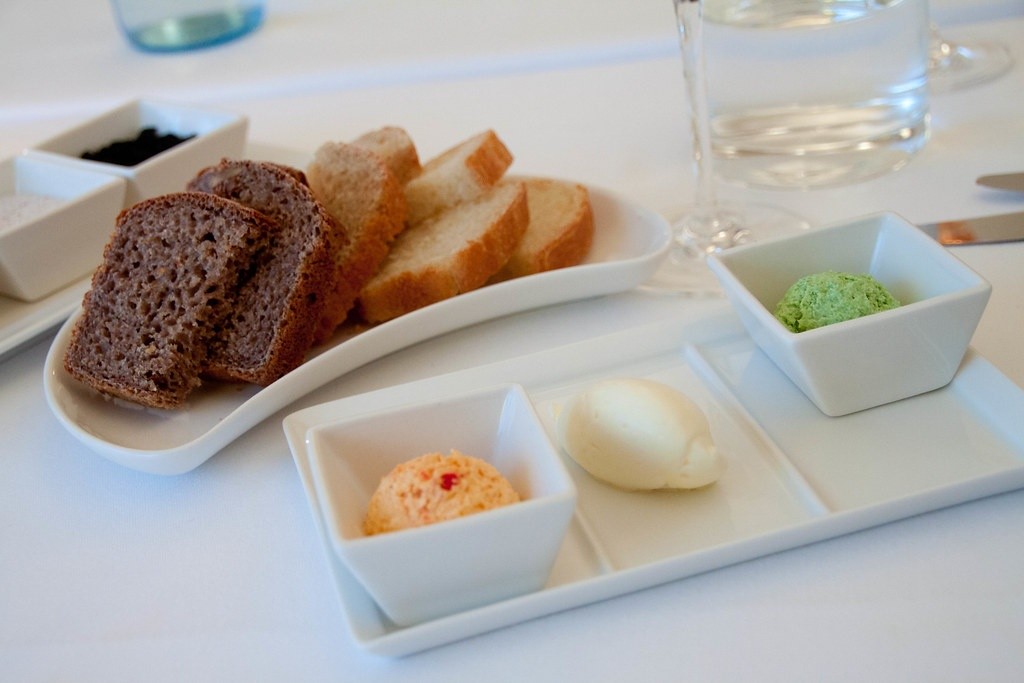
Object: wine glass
928 8 1015 96
636 0 814 292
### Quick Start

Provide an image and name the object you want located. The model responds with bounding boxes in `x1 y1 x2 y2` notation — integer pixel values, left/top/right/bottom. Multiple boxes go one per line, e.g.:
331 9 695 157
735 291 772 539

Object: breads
59 124 594 414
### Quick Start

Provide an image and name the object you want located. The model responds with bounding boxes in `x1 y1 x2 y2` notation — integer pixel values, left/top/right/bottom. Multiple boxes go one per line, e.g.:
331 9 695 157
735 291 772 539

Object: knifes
976 174 1024 192
913 211 1024 245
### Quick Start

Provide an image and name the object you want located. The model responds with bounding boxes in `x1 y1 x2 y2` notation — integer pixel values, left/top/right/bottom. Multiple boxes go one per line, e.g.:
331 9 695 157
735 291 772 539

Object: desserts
361 448 522 539
773 270 903 334
552 376 727 491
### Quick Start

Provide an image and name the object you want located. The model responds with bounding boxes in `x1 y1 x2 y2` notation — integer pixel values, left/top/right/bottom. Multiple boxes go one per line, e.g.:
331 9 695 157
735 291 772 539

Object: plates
44 183 673 476
0 272 100 356
281 303 1024 653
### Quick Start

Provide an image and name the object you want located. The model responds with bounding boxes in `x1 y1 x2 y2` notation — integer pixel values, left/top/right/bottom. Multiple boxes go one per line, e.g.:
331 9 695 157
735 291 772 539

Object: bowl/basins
0 151 130 302
705 210 993 418
305 381 577 627
22 98 250 205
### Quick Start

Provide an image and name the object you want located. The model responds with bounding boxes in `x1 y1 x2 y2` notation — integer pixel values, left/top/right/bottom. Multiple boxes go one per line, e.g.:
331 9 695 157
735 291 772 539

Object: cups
675 0 930 190
111 1 268 53
